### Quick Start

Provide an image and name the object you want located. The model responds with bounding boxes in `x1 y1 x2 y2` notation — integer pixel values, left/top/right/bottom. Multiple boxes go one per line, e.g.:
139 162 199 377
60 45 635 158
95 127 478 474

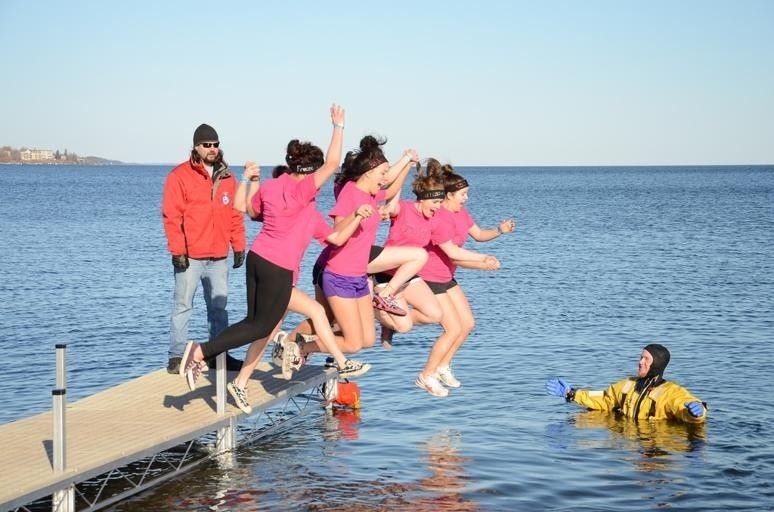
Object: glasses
196 141 220 148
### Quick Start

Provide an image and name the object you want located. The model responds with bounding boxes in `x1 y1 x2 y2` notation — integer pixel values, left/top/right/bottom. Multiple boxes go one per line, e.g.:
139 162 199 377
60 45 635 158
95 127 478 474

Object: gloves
685 401 704 418
545 378 568 397
172 255 189 269
233 251 246 268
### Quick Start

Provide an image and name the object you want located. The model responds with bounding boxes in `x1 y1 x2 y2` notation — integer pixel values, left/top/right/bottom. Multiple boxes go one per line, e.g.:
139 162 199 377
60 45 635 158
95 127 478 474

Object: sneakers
272 332 284 367
167 357 182 374
372 293 406 317
281 343 294 380
296 334 311 366
227 381 251 414
209 355 243 370
337 359 371 378
435 366 460 388
292 345 301 370
179 341 199 376
415 372 447 397
188 361 203 391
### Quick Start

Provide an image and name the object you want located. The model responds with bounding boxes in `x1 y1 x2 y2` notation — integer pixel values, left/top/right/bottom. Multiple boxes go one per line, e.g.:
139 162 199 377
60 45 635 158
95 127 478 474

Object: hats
193 124 218 145
643 344 671 378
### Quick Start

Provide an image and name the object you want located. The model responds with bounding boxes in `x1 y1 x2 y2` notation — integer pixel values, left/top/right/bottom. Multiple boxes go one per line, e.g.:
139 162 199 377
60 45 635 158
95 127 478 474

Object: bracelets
250 177 260 182
240 174 249 183
403 153 412 160
496 227 503 235
334 123 345 129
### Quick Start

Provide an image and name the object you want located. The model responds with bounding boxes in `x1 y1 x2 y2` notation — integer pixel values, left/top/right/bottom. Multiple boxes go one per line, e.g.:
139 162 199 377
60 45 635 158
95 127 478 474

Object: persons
544 343 707 425
373 157 501 349
272 149 429 368
281 135 421 381
415 164 515 399
162 123 247 375
225 160 372 415
178 102 345 391
